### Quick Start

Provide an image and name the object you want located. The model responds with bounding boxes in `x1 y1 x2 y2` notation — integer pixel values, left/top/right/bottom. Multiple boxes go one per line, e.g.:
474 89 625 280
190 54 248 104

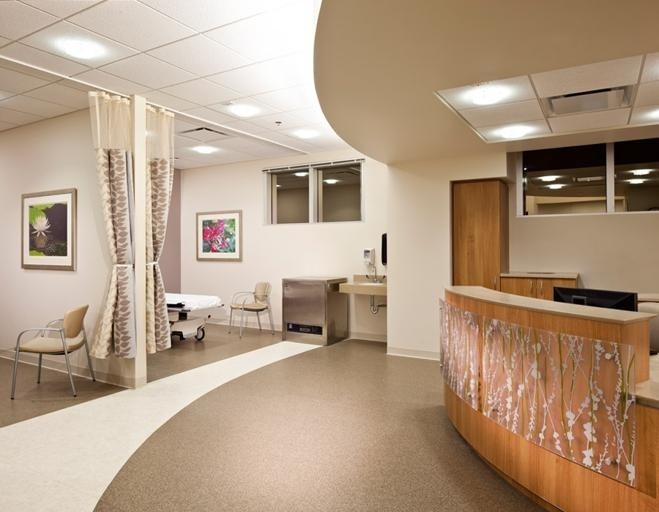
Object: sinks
358 281 383 286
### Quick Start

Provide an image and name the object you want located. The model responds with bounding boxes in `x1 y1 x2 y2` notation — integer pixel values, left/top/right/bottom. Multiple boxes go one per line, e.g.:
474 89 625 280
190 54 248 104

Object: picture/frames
21 188 77 271
197 211 243 261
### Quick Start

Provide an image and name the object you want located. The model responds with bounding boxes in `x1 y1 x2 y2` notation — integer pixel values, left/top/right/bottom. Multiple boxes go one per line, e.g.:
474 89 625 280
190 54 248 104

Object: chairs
229 282 275 339
10 304 96 401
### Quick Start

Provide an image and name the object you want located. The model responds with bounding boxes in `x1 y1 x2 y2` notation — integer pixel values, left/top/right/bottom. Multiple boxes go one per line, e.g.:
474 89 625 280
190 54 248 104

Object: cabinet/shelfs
454 181 509 291
500 279 577 300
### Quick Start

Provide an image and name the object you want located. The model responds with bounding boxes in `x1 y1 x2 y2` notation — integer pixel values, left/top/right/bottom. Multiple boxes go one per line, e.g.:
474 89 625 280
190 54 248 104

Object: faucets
372 266 377 282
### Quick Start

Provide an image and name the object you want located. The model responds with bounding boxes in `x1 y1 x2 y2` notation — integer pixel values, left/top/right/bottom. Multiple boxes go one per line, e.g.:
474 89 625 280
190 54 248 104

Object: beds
166 293 225 340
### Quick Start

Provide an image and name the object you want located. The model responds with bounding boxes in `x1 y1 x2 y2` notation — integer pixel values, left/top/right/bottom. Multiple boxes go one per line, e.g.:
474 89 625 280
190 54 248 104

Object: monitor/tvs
554 286 638 312
